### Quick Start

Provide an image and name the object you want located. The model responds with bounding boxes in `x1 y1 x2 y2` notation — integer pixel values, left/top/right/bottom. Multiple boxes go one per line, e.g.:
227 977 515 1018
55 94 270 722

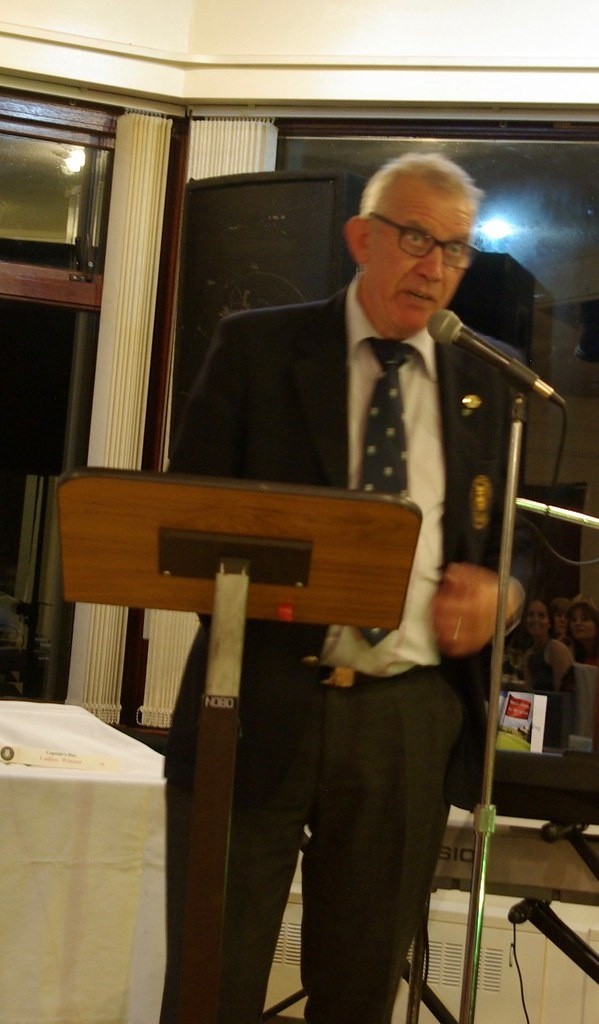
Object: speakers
170 167 368 463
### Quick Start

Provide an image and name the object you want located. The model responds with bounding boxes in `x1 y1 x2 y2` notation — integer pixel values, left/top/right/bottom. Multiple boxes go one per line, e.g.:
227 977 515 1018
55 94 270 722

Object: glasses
368 212 483 271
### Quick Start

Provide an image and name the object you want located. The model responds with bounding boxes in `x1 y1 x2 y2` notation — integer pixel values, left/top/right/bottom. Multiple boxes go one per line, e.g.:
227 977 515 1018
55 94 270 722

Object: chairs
560 664 599 750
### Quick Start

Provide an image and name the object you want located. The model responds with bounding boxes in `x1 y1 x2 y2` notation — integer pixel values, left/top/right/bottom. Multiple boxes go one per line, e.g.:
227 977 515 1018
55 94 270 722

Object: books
495 689 548 753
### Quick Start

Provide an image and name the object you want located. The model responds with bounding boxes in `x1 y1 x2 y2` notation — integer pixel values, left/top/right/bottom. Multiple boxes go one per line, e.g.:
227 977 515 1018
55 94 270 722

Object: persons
520 594 599 747
163 153 541 1024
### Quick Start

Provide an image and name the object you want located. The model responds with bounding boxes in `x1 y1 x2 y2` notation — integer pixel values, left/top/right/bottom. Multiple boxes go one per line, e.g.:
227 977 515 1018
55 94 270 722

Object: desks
0 699 167 1024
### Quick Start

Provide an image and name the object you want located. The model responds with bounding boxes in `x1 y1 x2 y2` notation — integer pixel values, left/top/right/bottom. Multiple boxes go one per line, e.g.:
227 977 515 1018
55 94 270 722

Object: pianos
408 823 599 906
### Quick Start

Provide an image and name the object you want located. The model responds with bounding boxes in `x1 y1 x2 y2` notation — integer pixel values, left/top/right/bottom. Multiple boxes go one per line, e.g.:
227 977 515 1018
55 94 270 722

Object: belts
315 665 429 689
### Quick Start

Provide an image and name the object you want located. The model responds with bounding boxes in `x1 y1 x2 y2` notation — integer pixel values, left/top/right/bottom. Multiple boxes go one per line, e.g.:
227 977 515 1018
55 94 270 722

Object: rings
452 632 458 641
454 616 462 632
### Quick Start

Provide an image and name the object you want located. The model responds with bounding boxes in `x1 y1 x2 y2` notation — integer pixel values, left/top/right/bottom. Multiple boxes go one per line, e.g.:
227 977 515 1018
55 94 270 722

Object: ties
356 333 412 648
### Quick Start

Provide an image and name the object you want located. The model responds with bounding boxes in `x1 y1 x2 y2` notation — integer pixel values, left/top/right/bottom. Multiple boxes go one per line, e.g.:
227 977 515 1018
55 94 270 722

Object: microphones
427 308 565 407
